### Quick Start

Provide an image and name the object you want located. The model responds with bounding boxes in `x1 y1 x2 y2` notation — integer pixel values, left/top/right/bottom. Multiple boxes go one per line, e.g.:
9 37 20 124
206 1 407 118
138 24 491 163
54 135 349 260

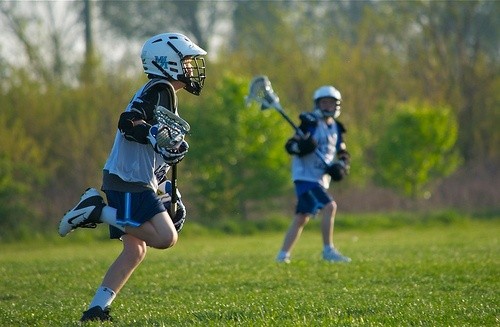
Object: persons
58 33 208 322
276 86 352 264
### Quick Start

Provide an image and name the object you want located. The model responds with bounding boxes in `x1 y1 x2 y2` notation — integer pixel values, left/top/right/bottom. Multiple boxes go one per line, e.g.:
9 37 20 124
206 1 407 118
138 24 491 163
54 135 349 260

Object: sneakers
323 247 351 263
57 187 108 237
80 305 114 322
275 255 290 264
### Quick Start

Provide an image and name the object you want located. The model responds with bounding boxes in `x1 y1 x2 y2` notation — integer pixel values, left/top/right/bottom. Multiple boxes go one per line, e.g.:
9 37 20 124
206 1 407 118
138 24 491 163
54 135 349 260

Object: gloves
159 180 186 233
295 132 317 158
148 124 189 166
328 160 346 182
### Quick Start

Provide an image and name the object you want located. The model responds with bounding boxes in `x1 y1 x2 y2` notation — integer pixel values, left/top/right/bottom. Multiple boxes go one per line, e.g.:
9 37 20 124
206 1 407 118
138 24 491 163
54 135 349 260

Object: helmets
313 86 341 118
141 33 207 96
